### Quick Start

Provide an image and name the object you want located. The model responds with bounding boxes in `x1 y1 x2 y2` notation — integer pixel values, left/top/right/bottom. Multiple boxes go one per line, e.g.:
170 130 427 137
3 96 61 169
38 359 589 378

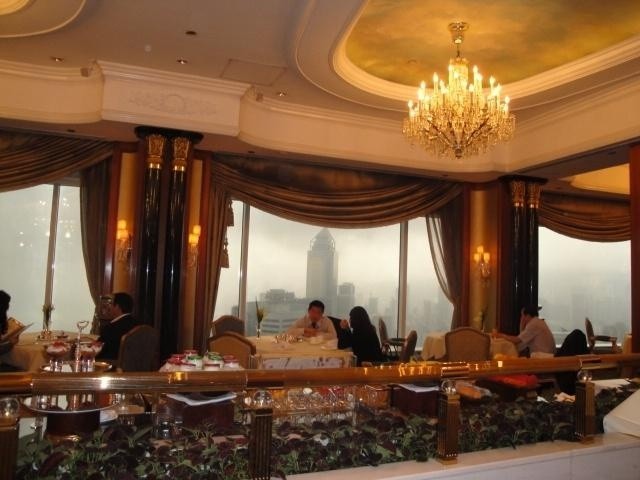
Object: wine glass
36 392 94 410
41 334 104 373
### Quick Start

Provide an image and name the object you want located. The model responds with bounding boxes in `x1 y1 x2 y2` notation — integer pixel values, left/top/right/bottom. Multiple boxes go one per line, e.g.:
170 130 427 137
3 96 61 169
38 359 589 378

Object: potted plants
254 294 265 340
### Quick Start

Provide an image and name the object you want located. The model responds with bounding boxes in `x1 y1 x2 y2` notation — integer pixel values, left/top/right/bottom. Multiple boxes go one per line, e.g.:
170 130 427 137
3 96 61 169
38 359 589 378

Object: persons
338 306 386 366
0 291 25 371
492 304 557 357
551 328 592 395
95 292 144 360
91 294 116 337
286 299 338 343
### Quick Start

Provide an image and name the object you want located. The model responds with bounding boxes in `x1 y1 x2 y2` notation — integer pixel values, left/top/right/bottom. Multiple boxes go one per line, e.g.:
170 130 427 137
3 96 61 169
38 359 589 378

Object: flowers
472 308 488 332
42 302 55 320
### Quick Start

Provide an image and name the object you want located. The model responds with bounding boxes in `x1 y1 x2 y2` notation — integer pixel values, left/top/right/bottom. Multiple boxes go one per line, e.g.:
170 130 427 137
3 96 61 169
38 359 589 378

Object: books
0 317 34 346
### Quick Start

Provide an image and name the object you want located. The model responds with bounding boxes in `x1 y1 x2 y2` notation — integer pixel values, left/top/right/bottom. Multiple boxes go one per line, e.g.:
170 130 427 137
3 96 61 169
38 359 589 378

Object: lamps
186 224 203 274
473 244 493 288
114 219 134 265
401 20 518 162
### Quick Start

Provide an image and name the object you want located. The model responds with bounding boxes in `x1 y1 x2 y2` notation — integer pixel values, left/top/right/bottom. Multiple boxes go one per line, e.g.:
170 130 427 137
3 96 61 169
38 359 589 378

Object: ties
311 322 316 328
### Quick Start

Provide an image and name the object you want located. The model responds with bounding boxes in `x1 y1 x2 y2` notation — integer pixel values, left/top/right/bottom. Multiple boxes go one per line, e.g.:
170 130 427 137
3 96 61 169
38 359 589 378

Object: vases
42 318 54 332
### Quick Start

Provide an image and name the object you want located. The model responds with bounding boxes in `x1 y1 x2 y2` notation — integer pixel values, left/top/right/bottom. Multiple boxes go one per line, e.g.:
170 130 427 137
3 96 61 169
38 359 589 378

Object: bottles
274 335 302 344
166 350 240 371
